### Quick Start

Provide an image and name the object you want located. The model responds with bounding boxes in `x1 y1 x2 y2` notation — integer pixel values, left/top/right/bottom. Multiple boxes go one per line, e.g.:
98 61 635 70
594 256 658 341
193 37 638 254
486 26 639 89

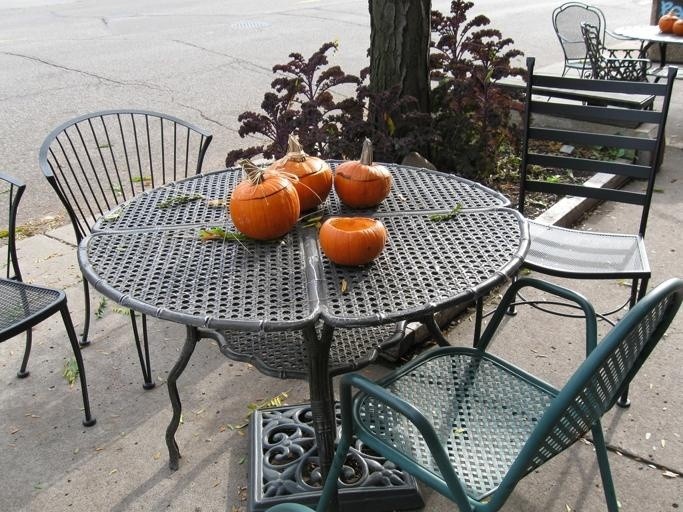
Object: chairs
317 277 683 509
545 2 643 104
34 104 219 393
464 57 679 407
1 170 102 431
580 20 654 108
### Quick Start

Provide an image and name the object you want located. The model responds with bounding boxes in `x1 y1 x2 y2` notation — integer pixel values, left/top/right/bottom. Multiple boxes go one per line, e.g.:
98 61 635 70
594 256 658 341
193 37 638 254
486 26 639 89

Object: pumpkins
271 134 333 210
659 12 679 33
319 217 386 265
229 158 300 241
673 20 683 35
333 139 391 208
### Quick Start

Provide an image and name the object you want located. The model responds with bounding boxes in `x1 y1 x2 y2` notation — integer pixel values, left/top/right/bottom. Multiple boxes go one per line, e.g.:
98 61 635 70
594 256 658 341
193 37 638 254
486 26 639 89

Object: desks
613 22 682 90
72 159 533 503
